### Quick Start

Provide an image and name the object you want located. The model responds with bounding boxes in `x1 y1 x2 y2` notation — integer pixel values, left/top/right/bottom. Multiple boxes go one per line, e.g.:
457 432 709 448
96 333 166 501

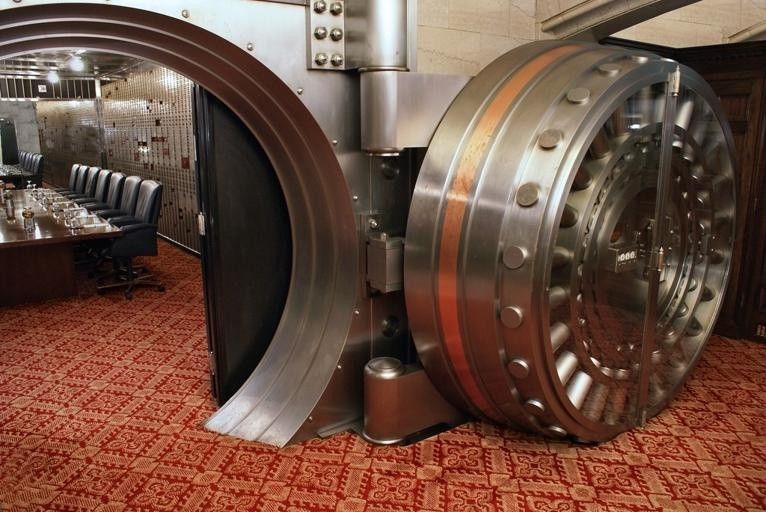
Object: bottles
22 206 35 217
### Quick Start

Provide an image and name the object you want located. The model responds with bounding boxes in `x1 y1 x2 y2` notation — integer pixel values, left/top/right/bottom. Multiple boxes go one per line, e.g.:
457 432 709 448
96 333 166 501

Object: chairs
0 150 165 306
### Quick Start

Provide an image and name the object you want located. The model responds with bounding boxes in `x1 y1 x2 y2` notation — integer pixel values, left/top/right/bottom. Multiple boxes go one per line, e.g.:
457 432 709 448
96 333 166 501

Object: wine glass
25 180 37 196
70 210 79 229
